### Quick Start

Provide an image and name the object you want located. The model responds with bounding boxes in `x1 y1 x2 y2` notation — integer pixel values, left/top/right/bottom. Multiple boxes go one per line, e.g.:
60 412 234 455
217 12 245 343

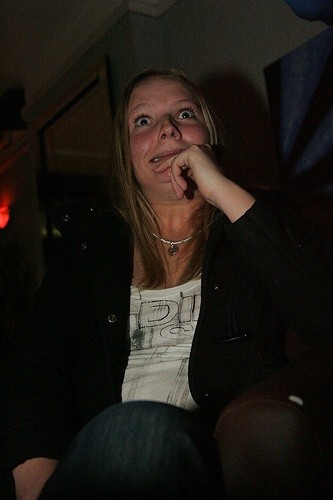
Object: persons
7 70 323 499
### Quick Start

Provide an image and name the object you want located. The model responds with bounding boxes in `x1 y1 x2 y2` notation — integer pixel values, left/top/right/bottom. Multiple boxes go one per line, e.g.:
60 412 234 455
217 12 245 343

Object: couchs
194 193 330 497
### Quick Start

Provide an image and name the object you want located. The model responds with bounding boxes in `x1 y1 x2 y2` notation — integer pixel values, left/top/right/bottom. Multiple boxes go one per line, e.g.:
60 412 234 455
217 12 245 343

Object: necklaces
150 229 200 255
